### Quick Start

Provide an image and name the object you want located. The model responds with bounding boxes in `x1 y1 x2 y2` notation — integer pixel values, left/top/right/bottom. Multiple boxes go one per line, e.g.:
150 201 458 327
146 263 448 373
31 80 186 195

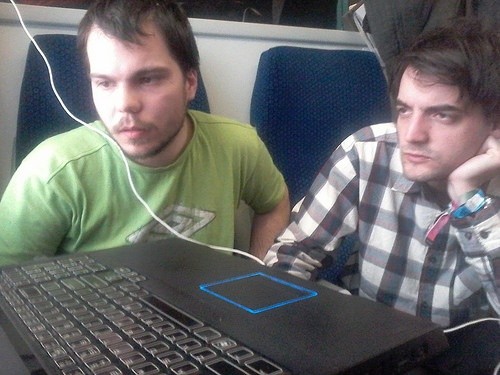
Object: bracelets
452 186 487 218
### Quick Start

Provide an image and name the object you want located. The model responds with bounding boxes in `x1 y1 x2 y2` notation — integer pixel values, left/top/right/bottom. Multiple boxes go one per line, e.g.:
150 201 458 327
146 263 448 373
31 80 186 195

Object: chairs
248 45 397 285
12 32 214 197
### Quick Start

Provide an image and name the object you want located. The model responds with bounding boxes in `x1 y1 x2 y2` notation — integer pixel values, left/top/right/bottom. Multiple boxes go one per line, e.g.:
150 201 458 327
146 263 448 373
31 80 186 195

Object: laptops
1 237 449 375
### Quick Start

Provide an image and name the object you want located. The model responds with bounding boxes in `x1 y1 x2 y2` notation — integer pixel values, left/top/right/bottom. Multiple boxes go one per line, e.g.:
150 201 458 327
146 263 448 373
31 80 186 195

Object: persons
255 18 500 375
0 0 293 274
